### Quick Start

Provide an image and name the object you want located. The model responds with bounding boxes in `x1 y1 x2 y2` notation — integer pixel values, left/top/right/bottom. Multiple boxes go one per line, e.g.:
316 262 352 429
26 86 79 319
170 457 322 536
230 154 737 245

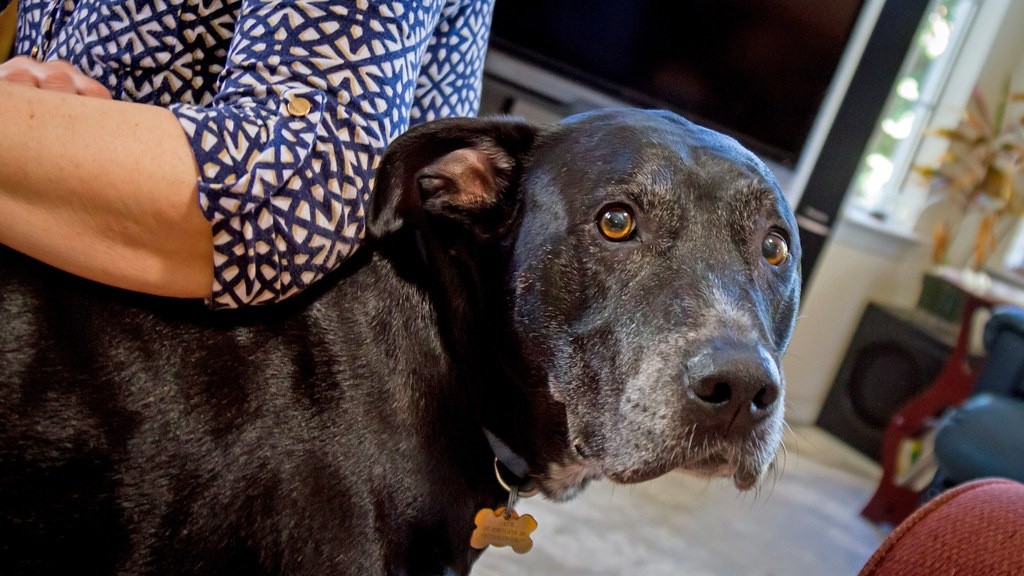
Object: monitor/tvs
468 0 932 307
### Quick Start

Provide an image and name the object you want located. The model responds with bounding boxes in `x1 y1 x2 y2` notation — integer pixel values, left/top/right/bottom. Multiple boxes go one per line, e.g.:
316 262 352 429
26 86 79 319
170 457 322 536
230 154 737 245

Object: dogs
1 104 805 576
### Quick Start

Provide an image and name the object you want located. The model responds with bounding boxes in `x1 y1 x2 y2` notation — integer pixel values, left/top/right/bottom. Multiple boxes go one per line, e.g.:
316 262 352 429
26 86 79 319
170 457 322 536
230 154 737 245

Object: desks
817 299 986 468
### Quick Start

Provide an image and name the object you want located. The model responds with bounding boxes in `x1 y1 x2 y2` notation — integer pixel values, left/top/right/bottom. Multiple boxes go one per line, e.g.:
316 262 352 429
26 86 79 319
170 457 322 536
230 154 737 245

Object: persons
0 0 494 310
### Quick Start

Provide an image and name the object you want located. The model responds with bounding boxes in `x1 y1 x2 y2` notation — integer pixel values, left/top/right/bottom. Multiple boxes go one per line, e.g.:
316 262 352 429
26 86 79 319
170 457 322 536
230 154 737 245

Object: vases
916 273 977 324
